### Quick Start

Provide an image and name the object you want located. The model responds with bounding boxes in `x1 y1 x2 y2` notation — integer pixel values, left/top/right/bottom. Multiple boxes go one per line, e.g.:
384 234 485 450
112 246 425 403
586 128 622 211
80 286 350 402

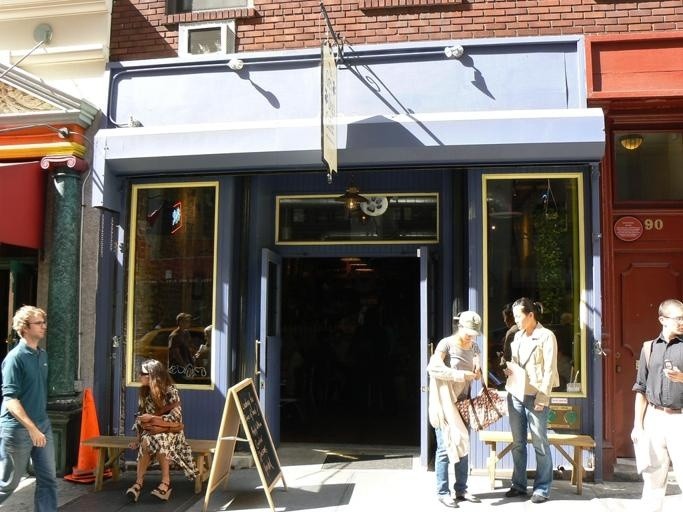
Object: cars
132 324 210 372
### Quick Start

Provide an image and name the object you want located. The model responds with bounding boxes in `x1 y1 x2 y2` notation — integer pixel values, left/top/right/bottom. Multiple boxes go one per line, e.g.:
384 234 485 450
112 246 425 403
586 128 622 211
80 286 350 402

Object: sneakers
439 496 458 508
456 491 481 503
532 493 547 502
505 489 525 496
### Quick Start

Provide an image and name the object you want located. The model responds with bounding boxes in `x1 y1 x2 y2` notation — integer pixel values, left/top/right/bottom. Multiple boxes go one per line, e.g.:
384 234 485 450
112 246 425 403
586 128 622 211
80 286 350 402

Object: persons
631 299 683 512
426 311 483 507
502 297 560 503
194 325 212 384
168 313 196 383
126 359 199 504
496 303 518 370
0 305 58 512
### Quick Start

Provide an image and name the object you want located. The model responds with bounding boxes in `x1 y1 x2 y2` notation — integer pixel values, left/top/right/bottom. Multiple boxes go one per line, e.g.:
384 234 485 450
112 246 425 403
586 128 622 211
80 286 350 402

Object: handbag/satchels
457 389 504 431
142 418 182 433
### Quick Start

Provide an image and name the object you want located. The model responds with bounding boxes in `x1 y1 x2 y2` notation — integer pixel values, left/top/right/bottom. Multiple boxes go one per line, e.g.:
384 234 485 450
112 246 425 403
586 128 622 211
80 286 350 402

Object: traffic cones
61 391 114 482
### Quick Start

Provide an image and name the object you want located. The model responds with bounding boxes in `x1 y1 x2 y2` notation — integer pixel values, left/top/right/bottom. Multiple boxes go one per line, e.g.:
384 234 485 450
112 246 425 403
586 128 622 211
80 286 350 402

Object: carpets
320 454 413 470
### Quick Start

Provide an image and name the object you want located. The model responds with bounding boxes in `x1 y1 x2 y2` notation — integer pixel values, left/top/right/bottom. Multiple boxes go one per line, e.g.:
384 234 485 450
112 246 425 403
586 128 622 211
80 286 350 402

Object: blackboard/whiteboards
237 382 284 491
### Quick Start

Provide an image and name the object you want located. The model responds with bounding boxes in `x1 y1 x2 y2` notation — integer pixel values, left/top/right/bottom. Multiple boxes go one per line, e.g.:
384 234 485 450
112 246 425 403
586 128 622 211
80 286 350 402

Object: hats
459 310 481 336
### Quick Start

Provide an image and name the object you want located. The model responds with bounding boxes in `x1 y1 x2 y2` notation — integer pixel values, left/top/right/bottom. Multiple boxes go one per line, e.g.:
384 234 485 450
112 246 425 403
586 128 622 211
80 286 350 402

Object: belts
651 404 682 414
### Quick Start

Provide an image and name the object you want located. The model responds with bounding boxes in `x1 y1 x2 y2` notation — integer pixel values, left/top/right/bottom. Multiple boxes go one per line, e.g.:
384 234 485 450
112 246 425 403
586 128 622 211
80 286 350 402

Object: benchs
479 430 594 496
81 435 217 495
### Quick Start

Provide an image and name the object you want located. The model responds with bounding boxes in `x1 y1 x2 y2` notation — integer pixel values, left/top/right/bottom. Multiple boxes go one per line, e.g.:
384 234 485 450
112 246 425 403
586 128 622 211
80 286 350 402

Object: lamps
619 135 644 150
335 186 369 209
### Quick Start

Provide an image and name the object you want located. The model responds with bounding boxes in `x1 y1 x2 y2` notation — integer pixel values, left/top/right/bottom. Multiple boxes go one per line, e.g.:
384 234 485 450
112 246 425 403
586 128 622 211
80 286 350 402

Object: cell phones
664 360 673 370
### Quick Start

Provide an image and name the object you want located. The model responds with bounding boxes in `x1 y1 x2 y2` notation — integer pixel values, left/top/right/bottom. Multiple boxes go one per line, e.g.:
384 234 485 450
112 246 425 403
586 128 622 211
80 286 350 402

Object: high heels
126 482 141 502
151 482 171 500
